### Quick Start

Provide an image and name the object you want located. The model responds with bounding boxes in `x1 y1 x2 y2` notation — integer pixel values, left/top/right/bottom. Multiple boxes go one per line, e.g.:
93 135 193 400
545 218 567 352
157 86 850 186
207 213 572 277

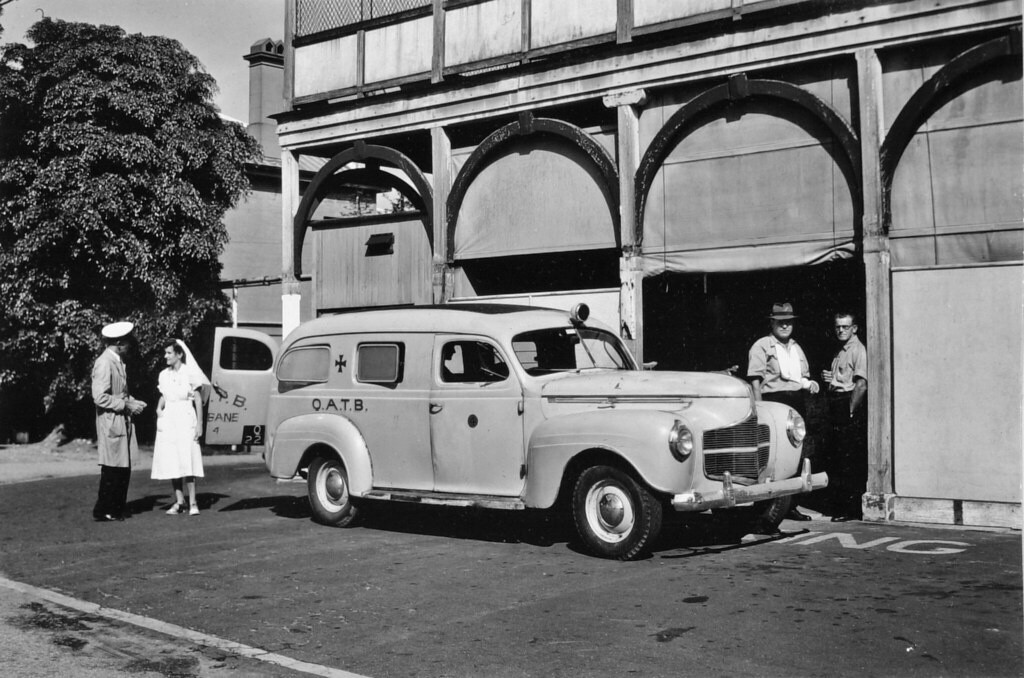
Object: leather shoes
830 511 849 522
782 507 812 521
92 511 125 521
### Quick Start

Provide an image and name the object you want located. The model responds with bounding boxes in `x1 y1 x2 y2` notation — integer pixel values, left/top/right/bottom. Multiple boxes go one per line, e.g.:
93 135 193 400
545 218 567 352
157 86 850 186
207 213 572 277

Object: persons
148 336 210 516
746 301 820 535
89 321 148 522
821 310 869 523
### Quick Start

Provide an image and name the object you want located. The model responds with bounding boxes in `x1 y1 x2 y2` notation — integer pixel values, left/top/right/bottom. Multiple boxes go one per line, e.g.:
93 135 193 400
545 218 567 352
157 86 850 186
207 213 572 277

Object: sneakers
164 502 185 514
189 502 200 515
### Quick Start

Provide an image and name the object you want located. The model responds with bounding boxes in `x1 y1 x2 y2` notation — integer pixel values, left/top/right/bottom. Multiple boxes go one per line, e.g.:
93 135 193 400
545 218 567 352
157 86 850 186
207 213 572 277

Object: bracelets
849 412 854 415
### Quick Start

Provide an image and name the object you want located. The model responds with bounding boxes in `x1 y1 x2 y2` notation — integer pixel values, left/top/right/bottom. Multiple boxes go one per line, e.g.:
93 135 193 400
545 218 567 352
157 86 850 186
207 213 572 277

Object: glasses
834 324 854 330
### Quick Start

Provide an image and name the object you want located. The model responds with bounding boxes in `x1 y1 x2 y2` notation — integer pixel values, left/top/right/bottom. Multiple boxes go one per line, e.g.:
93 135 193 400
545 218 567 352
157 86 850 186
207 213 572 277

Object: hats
768 302 800 319
101 321 134 338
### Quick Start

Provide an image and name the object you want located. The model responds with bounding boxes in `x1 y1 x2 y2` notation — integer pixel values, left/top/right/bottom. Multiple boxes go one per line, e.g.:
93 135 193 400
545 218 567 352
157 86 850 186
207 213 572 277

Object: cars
202 302 830 562
